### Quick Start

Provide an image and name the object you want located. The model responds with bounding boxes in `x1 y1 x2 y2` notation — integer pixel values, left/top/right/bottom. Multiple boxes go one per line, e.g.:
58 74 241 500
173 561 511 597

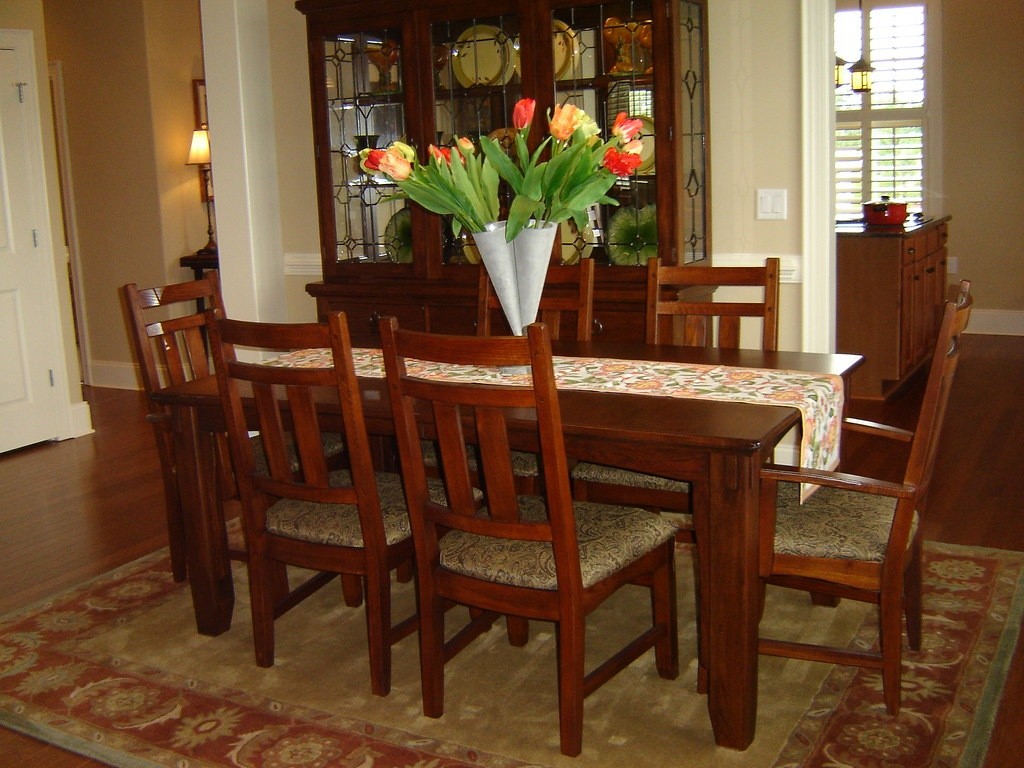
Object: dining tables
151 335 864 752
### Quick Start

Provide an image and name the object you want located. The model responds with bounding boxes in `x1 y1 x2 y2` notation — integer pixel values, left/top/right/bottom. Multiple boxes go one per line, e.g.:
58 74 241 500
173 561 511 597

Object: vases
471 220 522 338
514 220 557 336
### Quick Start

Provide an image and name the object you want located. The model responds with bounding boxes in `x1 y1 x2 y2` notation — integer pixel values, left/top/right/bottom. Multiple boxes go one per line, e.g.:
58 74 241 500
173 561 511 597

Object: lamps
835 56 848 89
185 129 217 257
848 0 875 94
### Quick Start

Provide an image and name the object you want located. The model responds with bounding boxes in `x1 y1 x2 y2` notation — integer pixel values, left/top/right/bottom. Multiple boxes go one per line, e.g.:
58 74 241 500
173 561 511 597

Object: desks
179 253 219 360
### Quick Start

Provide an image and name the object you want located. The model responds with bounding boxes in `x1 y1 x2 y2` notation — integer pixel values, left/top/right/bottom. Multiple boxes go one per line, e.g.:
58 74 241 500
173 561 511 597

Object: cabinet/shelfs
835 215 954 401
294 0 713 277
303 280 718 346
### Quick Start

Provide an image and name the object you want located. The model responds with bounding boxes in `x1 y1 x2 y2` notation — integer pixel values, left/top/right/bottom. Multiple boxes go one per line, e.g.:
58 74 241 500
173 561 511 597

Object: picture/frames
190 77 214 205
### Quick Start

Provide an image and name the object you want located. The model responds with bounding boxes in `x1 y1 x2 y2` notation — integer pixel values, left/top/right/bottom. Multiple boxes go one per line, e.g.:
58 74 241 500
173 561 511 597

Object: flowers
479 96 642 244
357 136 503 239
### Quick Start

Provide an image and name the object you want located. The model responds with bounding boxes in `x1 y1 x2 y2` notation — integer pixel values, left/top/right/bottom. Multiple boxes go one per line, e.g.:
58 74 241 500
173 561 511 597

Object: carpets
0 517 1023 768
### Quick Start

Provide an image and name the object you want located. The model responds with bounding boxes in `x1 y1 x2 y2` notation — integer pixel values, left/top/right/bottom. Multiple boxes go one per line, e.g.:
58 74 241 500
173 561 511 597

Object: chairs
376 314 680 757
759 279 975 715
114 271 360 601
571 256 782 546
394 257 592 501
204 304 493 697
